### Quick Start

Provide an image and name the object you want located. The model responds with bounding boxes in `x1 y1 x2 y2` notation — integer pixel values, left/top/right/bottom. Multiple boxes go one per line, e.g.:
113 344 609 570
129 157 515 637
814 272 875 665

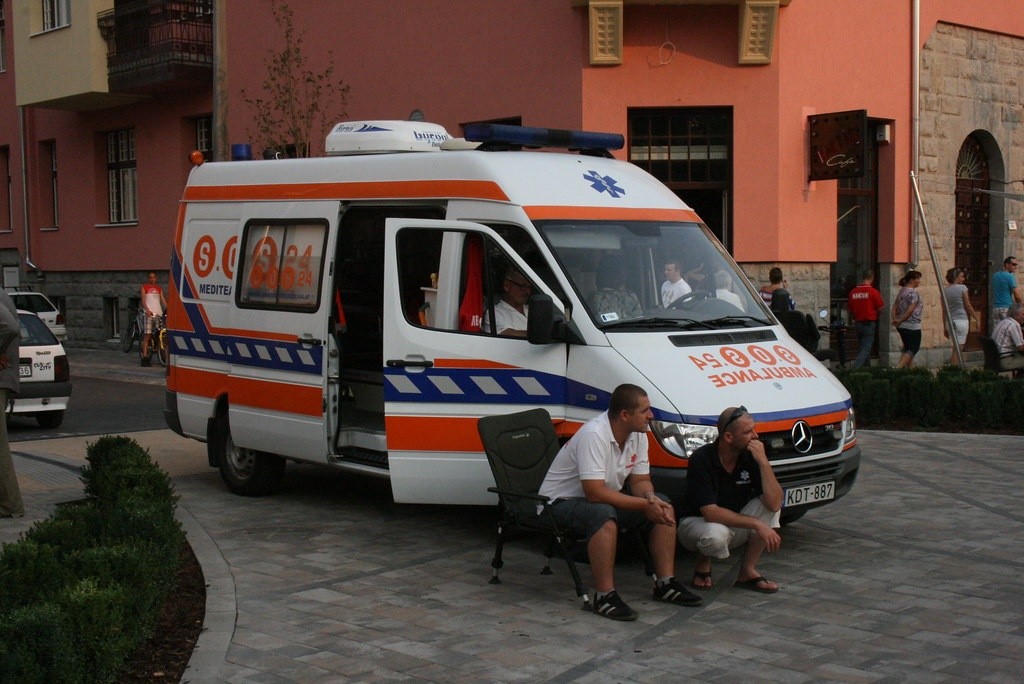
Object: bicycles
123 306 167 367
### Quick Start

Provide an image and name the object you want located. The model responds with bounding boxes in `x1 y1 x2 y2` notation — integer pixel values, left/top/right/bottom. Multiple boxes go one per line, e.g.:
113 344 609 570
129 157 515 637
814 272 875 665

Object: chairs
477 409 657 611
975 335 1023 377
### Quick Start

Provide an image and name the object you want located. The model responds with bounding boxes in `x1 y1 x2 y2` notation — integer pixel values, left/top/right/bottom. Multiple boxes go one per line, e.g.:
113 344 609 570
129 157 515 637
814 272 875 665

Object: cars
6 309 72 429
6 292 68 346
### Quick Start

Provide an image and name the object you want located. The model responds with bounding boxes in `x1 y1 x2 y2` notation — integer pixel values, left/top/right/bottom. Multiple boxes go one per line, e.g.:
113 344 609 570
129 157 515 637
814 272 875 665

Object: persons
892 271 923 369
758 267 796 310
674 407 785 593
849 269 887 370
537 384 703 621
714 269 745 314
940 267 979 365
990 256 1022 319
991 304 1024 370
484 266 530 336
0 287 27 519
661 259 692 309
141 271 167 367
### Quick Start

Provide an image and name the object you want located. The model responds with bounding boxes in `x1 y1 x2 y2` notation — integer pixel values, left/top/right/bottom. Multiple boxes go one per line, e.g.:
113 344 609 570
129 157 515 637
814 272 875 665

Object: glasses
723 406 747 432
957 268 961 273
508 279 533 288
1007 262 1017 266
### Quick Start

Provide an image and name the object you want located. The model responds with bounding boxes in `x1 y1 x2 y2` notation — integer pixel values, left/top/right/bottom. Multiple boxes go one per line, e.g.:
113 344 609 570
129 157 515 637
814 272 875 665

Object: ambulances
167 122 860 524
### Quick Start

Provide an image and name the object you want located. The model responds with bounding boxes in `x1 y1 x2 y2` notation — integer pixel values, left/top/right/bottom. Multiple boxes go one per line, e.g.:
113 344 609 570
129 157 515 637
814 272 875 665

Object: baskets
161 316 167 328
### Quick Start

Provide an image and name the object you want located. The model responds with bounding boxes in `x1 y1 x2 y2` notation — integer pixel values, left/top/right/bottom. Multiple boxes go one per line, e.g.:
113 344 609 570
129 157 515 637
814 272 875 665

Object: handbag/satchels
779 310 820 354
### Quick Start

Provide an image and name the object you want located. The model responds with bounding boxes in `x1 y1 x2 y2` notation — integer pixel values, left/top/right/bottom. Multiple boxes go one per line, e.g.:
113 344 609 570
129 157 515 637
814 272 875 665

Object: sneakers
653 577 703 606
593 590 639 620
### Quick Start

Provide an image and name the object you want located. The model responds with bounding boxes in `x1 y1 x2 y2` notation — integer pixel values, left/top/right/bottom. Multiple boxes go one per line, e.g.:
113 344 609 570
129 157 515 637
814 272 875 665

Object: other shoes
11 510 25 518
142 357 149 367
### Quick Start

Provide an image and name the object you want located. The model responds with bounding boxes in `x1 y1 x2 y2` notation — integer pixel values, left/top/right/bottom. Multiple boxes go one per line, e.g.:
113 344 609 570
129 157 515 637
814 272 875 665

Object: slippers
692 568 713 590
736 576 778 593
